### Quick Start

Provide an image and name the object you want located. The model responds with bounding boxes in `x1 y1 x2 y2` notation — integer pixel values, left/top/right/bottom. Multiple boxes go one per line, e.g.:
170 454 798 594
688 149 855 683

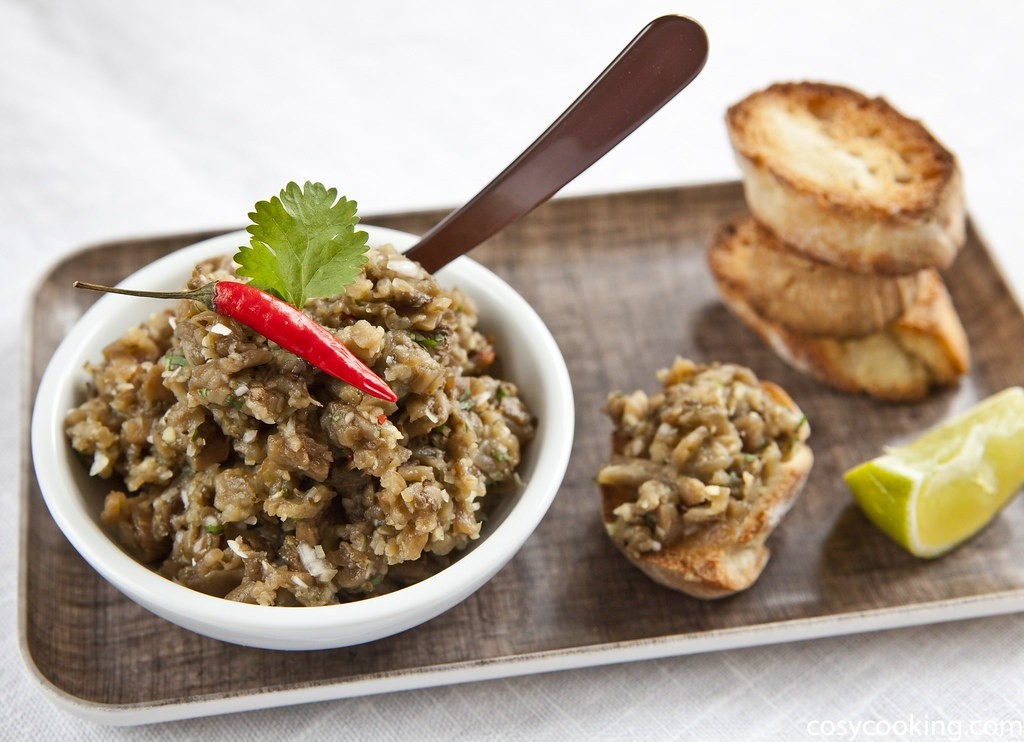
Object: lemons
841 385 1024 559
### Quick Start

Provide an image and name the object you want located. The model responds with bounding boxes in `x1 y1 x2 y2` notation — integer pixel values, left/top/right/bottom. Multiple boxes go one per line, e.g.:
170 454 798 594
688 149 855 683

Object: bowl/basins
33 224 575 650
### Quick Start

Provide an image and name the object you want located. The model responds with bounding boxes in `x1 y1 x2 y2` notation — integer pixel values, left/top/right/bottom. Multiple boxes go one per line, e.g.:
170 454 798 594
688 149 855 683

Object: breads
709 80 963 400
599 377 816 599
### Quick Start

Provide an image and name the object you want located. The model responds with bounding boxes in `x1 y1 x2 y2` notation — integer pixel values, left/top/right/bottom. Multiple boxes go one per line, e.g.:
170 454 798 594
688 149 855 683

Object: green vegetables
233 180 371 309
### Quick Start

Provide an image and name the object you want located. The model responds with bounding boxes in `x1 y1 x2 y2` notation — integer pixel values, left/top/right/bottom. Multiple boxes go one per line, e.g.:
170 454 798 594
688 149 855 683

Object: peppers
71 281 397 403
